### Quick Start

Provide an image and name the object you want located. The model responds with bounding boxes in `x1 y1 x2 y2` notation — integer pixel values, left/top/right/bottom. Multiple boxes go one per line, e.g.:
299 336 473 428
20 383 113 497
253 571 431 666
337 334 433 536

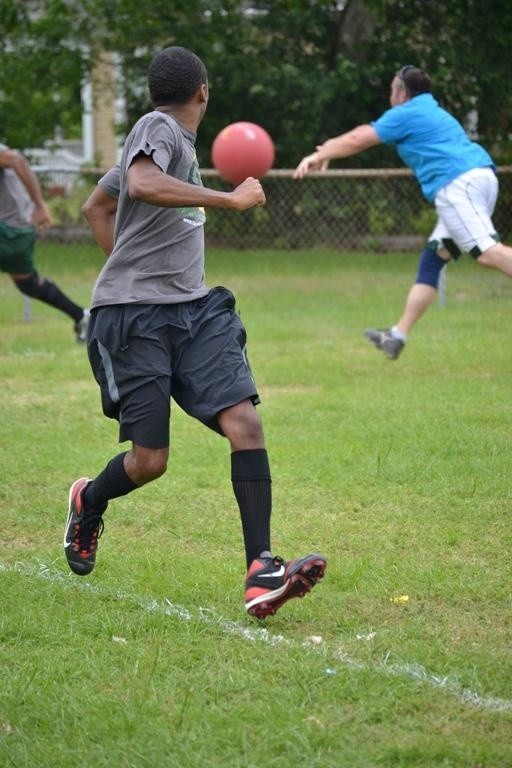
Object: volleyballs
212 120 273 186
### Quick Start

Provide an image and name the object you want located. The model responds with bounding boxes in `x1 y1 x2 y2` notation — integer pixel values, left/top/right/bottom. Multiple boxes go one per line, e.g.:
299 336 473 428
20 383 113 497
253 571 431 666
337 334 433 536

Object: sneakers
362 326 406 362
62 475 106 577
74 306 93 342
241 549 329 621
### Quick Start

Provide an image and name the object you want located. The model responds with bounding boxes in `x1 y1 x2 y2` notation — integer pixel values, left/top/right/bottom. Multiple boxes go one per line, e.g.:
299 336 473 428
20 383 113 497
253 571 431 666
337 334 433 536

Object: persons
0 142 91 344
62 43 328 622
291 64 512 361
